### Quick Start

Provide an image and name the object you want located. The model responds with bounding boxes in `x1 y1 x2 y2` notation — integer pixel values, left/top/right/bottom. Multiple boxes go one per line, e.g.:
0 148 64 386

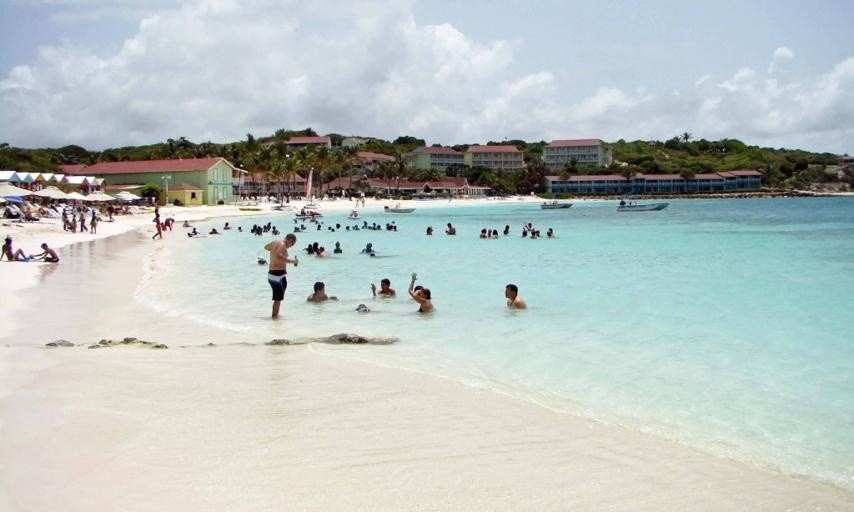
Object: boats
383 206 416 214
346 210 362 220
540 201 574 209
615 199 670 212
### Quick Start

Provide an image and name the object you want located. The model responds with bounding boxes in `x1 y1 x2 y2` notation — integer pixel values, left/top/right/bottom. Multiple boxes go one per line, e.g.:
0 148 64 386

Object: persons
504 283 526 310
479 223 553 239
427 223 455 235
0 236 31 262
295 199 397 257
369 277 396 297
139 197 199 240
409 272 422 299
6 202 130 234
304 282 338 302
263 232 298 320
412 287 433 313
210 222 278 235
29 243 60 264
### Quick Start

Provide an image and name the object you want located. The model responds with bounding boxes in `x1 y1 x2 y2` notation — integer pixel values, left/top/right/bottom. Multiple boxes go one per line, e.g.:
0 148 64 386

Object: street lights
161 176 171 205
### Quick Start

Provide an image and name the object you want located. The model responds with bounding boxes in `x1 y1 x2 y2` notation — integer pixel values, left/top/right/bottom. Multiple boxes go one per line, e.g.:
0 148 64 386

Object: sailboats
236 154 324 219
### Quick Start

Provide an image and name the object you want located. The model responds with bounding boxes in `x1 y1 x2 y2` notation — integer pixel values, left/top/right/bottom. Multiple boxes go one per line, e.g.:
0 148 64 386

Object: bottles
293 255 298 267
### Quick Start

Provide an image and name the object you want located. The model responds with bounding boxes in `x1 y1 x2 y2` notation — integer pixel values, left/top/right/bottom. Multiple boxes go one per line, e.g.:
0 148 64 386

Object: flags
305 167 314 200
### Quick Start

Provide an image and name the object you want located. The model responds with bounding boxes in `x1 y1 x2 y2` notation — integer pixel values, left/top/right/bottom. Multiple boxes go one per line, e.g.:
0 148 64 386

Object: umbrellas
31 186 69 203
0 181 35 202
115 189 141 202
85 191 117 202
63 190 87 202
